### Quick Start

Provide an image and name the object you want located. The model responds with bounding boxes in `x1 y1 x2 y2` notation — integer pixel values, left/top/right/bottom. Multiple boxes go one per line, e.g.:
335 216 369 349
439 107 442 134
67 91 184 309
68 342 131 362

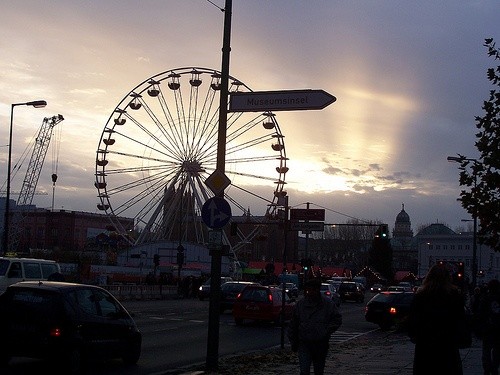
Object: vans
0 257 62 291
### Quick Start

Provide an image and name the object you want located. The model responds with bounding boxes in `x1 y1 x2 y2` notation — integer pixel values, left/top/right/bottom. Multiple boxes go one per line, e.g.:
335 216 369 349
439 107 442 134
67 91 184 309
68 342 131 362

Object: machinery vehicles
1 113 64 259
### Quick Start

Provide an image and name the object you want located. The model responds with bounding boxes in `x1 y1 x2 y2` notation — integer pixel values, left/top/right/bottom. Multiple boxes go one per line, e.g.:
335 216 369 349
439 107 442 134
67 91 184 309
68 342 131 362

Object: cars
233 285 298 325
364 290 421 328
197 277 234 301
0 280 142 375
270 271 370 303
371 283 387 292
388 281 423 294
220 281 262 314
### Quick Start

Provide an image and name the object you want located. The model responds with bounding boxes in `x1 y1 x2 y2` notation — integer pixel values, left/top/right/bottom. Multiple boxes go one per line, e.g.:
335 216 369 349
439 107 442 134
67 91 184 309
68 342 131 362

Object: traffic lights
380 225 388 239
304 258 309 272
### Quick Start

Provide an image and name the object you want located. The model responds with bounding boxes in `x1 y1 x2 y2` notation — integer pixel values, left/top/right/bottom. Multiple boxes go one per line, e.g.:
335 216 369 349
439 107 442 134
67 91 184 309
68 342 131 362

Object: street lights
446 154 478 292
3 99 48 255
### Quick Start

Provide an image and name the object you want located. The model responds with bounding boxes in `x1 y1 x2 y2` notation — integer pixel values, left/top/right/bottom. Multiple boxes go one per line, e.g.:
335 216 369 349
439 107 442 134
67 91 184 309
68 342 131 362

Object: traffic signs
229 88 336 112
289 208 326 221
289 221 324 232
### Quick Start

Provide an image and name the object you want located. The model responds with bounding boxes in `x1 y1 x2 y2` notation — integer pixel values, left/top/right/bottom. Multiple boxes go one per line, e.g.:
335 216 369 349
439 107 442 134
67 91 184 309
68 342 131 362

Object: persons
406 261 500 375
288 279 342 375
